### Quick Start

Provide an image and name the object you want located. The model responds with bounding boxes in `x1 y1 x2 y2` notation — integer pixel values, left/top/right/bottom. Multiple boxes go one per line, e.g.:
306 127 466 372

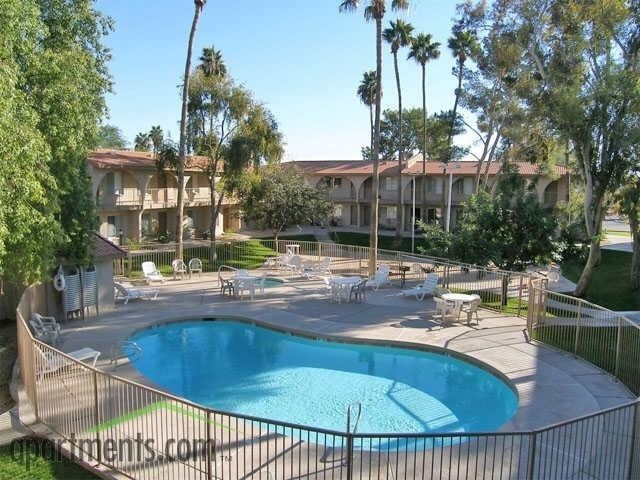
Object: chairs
216 252 483 328
28 310 101 385
111 255 205 305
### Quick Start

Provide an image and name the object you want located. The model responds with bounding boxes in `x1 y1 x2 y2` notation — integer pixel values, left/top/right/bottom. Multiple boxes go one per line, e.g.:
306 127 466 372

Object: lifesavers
53 273 65 291
